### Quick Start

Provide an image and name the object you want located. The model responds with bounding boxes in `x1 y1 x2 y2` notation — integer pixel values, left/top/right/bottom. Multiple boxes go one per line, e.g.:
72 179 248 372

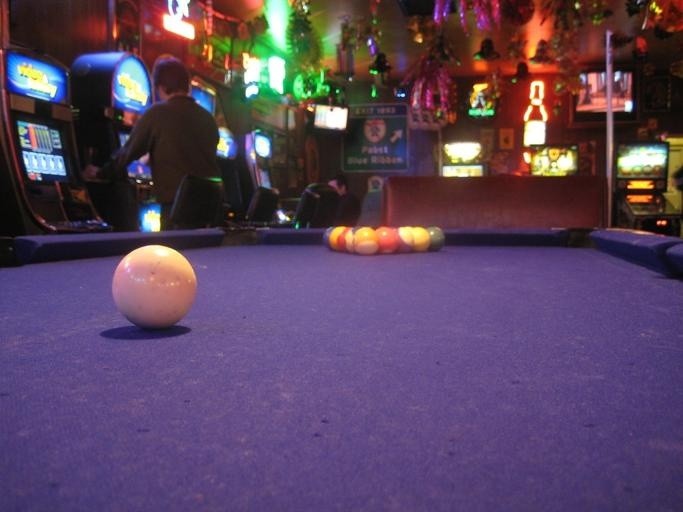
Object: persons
328 174 360 226
82 54 220 232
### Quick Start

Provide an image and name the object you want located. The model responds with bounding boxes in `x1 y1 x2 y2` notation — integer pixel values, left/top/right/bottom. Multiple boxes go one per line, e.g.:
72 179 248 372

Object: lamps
368 0 555 79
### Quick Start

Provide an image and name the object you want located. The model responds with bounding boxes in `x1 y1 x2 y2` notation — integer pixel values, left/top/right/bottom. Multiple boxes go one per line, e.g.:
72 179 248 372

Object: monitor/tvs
312 104 349 132
119 130 154 183
16 114 70 186
257 168 274 192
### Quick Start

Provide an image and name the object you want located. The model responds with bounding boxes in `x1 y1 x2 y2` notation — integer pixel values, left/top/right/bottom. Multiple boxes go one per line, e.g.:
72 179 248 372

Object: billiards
322 225 445 255
111 244 198 329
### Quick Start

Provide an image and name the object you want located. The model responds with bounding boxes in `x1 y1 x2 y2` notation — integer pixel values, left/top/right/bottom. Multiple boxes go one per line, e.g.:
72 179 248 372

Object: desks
1 223 681 511
